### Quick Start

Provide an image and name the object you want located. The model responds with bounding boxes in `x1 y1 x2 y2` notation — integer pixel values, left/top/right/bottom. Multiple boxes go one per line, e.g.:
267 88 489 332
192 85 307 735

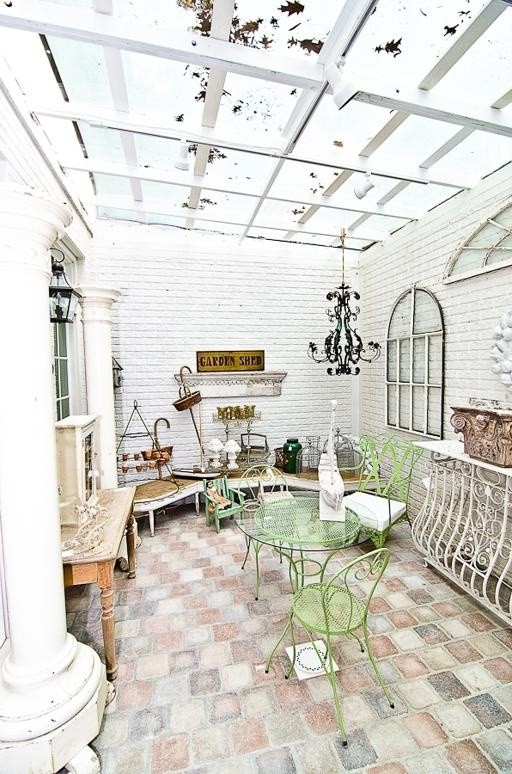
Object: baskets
274 446 285 468
173 386 201 411
141 444 173 466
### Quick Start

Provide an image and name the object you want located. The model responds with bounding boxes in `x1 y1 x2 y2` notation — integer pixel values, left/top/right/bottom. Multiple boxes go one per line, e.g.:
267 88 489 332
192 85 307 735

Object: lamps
305 226 382 376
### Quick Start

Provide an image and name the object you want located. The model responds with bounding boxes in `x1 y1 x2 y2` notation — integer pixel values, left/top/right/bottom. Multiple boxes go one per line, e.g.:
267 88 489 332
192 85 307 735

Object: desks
60 487 146 681
231 488 378 675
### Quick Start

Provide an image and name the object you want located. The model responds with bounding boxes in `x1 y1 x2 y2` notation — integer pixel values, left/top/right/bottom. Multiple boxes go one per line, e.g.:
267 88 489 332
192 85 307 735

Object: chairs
234 462 300 602
338 431 426 559
203 433 426 540
262 546 397 747
